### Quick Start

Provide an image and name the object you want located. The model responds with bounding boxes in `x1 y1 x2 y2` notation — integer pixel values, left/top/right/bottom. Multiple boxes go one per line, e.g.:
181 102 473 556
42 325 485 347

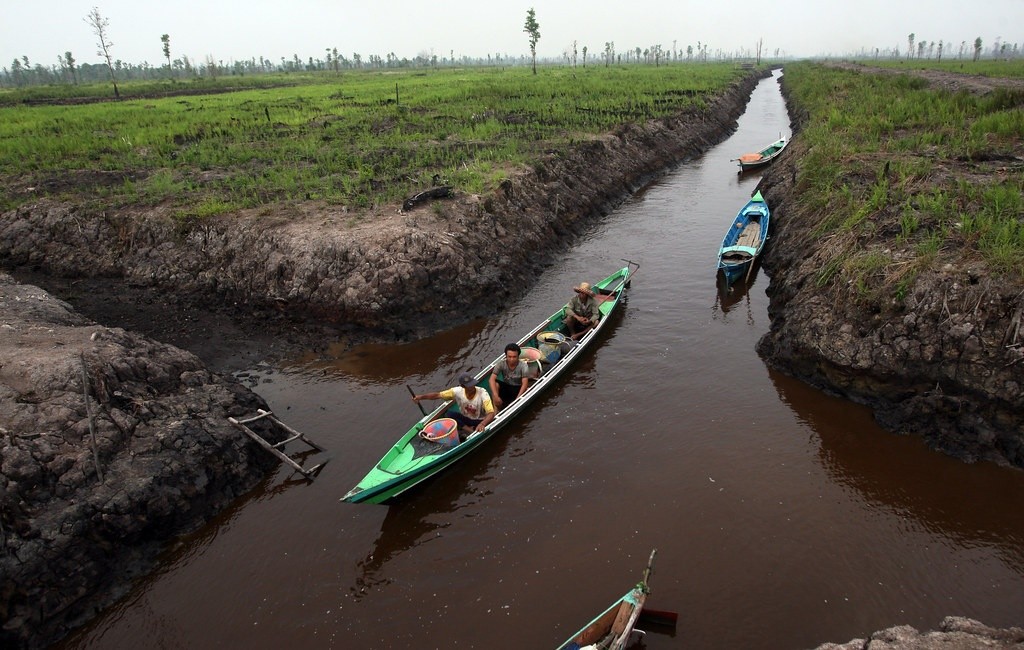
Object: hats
458 373 480 387
573 282 596 298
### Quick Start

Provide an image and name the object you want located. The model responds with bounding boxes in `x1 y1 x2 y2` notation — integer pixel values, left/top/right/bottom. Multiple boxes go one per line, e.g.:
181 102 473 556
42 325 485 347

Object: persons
565 282 600 341
489 343 529 416
412 372 494 432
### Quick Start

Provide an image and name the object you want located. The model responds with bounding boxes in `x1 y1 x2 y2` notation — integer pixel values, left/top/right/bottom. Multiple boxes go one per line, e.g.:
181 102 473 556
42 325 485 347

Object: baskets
536 331 565 363
519 346 543 379
420 417 461 447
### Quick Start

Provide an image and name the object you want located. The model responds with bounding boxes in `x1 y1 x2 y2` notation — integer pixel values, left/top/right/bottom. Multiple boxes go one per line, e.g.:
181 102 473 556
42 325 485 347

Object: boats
555 546 659 650
717 189 770 290
738 135 788 174
338 257 641 505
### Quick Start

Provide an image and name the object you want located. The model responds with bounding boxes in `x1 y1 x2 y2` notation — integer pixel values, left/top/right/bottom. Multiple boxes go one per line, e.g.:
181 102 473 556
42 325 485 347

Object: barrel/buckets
517 347 542 378
422 418 461 447
536 331 565 364
